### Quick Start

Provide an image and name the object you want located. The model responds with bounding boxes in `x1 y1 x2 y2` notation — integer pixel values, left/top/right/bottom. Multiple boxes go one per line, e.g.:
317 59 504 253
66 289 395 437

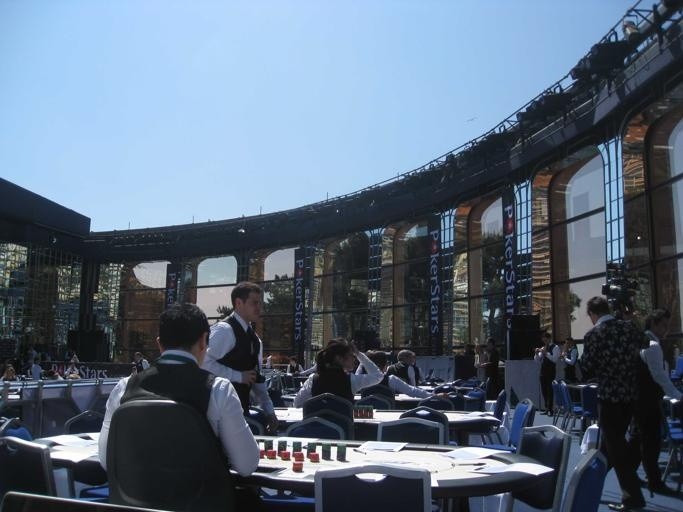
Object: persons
579 296 649 511
0 344 79 380
266 353 275 369
533 333 578 416
131 366 137 376
638 308 681 495
200 280 278 435
98 301 260 476
386 350 425 387
354 350 447 409
472 338 499 401
134 351 149 374
293 337 384 409
286 357 317 376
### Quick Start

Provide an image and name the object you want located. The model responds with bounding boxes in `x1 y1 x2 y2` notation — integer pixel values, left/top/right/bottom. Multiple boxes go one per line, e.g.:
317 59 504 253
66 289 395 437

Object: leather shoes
540 410 553 416
608 503 643 511
647 479 672 495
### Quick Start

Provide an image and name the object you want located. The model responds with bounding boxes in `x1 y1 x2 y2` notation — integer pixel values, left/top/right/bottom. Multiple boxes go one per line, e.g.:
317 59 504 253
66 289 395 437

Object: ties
247 326 252 342
